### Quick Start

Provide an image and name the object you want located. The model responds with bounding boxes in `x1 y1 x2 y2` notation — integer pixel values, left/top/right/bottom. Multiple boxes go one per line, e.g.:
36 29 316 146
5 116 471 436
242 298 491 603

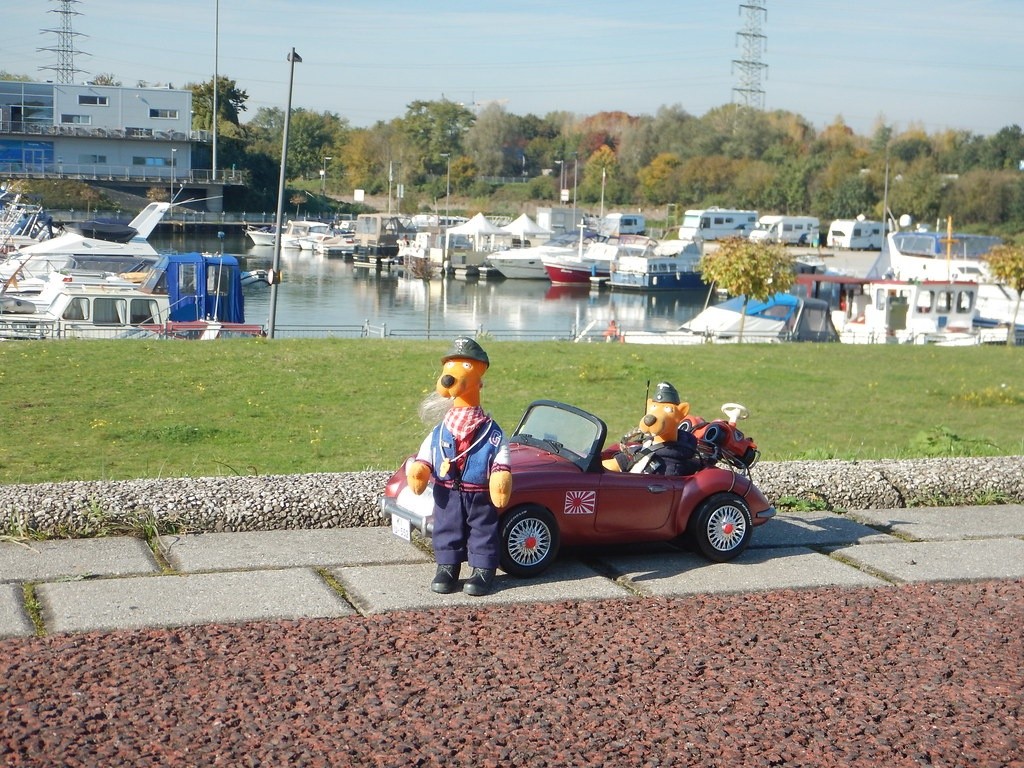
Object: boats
1 205 1023 347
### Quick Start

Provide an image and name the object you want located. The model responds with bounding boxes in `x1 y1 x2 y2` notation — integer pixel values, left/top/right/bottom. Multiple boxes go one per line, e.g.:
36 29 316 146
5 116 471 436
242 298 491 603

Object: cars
377 376 778 580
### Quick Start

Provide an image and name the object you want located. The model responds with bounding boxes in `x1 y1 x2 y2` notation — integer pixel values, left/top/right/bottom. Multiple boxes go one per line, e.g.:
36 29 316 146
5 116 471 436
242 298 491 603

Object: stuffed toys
601 380 699 476
406 335 513 597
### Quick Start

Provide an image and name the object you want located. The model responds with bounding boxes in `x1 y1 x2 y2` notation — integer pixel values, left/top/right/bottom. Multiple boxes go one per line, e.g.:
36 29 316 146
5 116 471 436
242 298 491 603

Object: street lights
264 47 303 339
